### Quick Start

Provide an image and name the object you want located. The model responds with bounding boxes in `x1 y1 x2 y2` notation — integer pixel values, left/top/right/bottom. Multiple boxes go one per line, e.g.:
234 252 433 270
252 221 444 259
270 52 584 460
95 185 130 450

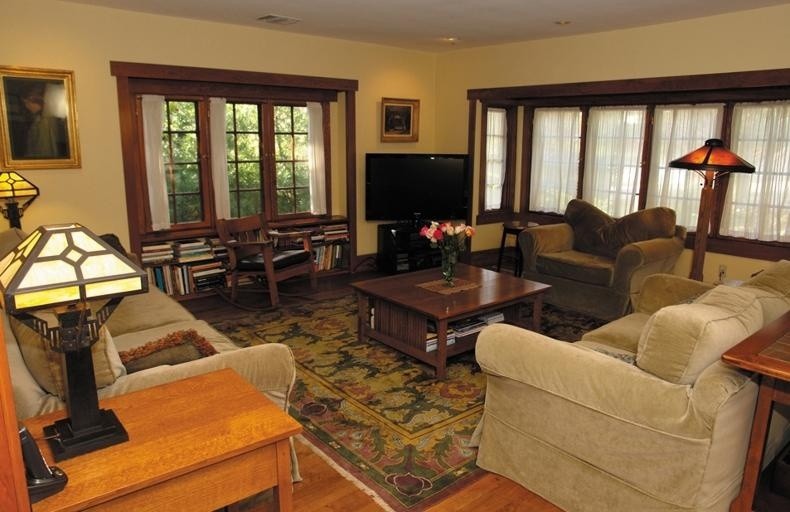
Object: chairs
215 210 320 309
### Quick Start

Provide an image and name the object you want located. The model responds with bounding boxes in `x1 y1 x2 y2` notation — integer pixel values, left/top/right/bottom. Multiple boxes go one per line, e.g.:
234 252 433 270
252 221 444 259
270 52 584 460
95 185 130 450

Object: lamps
1 220 155 463
668 135 759 285
0 165 41 232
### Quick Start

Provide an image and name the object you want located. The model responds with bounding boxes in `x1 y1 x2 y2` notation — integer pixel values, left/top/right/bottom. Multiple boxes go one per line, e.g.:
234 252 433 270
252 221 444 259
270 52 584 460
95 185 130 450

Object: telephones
713 265 727 286
18 421 67 504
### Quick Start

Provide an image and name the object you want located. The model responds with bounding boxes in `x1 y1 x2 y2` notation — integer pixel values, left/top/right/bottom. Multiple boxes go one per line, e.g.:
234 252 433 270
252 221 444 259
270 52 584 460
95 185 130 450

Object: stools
495 219 541 279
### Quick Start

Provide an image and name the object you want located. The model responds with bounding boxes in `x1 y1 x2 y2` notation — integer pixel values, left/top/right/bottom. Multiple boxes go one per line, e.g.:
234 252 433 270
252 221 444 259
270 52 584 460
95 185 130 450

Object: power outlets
716 263 727 276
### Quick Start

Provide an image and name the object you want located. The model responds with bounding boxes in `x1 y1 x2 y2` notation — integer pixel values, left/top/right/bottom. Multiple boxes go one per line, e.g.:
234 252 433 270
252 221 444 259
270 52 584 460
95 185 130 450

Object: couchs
469 253 789 512
514 196 689 328
1 224 303 505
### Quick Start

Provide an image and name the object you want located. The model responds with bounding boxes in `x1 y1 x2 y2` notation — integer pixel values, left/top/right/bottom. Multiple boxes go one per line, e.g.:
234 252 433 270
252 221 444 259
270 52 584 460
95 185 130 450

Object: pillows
3 300 129 400
113 325 220 377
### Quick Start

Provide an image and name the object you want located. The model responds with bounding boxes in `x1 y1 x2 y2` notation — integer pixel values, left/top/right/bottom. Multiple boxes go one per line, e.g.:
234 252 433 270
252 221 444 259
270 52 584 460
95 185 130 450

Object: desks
11 363 303 511
720 311 790 512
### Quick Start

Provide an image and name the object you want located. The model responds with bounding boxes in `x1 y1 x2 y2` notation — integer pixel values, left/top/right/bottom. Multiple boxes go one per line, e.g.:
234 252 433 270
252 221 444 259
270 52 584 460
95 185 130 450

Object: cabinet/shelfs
132 213 349 301
375 222 443 277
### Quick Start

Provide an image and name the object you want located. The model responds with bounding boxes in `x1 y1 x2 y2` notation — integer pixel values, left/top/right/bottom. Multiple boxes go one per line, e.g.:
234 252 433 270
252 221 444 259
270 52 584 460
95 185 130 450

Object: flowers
417 218 478 280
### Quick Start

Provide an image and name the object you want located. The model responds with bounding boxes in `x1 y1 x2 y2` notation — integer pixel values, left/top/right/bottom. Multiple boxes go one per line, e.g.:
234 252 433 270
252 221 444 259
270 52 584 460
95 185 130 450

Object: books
139 220 349 298
369 304 509 354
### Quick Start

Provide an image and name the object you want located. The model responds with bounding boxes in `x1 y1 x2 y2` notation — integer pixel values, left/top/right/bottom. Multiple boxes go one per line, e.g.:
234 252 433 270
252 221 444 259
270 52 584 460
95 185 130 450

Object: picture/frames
378 95 421 144
0 65 81 172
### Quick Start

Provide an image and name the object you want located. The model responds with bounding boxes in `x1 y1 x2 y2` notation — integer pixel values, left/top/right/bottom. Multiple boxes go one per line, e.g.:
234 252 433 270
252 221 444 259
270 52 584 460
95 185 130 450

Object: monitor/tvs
365 152 473 227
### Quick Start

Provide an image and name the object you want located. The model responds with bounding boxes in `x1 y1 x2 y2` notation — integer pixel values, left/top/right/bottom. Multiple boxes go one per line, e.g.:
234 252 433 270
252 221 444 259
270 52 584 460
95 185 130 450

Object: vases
436 244 465 289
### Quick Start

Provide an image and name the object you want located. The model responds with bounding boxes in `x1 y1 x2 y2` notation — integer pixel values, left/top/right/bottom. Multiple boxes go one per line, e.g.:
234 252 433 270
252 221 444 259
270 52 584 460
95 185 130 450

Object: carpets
199 292 612 512
